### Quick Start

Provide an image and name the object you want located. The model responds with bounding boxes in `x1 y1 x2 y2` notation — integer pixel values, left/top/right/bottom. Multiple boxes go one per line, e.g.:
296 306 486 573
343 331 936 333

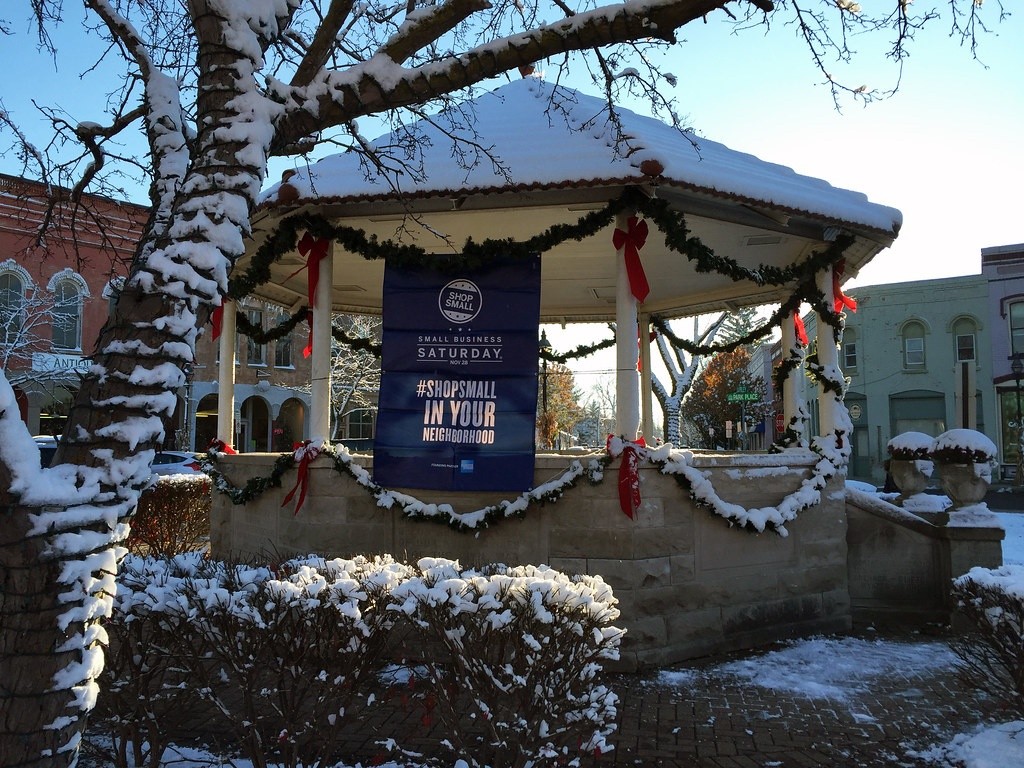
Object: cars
31 434 66 451
151 449 220 479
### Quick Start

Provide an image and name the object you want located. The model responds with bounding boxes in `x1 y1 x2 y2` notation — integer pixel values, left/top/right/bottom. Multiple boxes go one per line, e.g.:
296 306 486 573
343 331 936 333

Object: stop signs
775 414 785 432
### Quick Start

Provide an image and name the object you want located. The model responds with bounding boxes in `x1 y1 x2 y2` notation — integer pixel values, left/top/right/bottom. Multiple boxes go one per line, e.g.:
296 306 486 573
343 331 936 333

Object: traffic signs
727 392 759 401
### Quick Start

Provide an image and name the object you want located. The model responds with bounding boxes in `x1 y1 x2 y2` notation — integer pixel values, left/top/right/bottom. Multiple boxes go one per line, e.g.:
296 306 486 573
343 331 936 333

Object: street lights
1011 350 1023 487
540 328 552 413
708 423 714 450
